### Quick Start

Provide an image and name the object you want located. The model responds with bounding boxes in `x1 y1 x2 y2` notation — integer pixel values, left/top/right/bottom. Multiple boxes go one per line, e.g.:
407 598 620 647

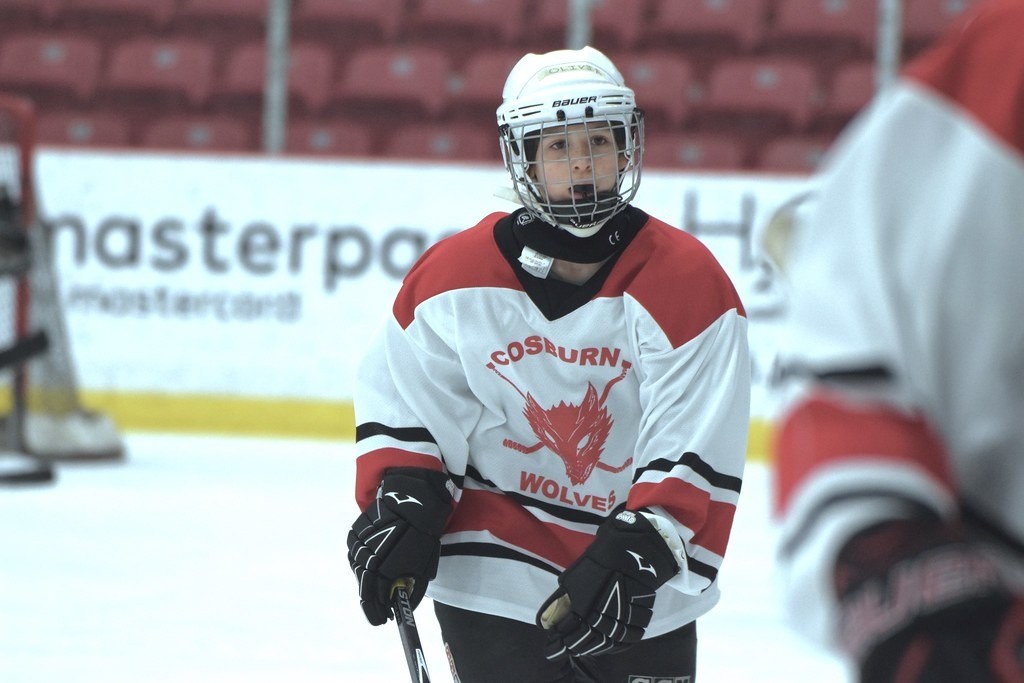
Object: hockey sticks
389 581 432 682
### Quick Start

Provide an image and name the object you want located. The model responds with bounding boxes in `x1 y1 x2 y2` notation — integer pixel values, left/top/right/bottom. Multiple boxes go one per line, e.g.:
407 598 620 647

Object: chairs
0 0 953 178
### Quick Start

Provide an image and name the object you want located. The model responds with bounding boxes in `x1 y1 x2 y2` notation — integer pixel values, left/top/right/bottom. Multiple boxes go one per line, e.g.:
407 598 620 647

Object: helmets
496 46 645 238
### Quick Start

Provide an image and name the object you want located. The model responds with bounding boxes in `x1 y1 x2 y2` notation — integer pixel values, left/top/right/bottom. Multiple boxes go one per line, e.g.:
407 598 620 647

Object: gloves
833 509 1023 680
347 466 455 627
536 509 680 664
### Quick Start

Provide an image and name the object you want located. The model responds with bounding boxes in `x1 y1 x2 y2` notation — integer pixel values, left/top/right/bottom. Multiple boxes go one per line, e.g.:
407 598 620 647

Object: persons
346 45 751 683
765 0 1024 683
